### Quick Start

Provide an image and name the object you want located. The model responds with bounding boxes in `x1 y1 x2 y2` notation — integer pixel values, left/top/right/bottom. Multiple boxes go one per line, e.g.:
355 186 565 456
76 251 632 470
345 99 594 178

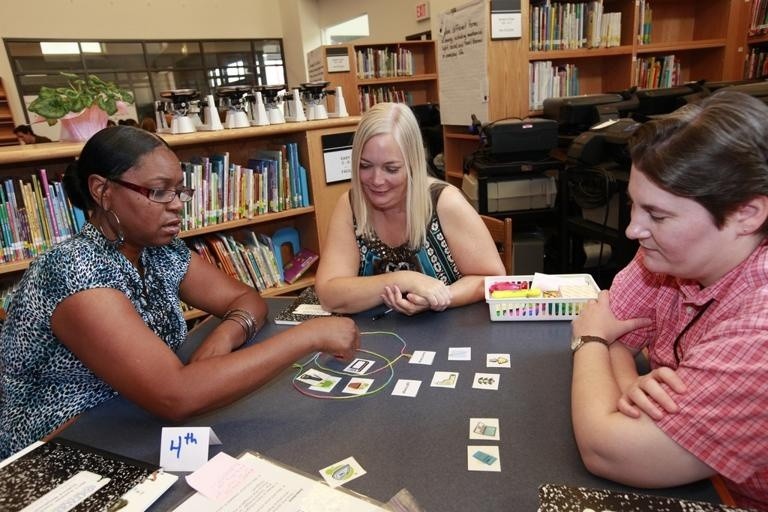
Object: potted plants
34 70 134 140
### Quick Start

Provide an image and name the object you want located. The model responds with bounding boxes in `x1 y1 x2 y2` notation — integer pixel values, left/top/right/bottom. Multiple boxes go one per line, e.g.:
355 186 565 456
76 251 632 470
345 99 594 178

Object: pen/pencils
371 307 397 322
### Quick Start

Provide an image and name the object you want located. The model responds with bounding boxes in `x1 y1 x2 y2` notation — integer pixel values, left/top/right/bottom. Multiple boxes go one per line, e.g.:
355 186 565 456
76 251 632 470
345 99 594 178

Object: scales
154 80 350 135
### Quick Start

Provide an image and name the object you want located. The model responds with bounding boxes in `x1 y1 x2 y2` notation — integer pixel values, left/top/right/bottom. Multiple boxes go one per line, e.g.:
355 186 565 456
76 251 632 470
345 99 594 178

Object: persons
315 102 506 316
571 91 768 512
0 124 362 463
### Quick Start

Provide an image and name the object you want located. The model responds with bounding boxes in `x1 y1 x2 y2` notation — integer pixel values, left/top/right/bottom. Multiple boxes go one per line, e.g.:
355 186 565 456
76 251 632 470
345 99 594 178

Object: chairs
478 216 513 277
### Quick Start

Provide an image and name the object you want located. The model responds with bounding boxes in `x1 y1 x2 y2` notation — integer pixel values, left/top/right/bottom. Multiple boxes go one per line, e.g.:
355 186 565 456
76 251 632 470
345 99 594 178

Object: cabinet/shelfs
1 115 363 334
306 42 438 119
489 0 768 126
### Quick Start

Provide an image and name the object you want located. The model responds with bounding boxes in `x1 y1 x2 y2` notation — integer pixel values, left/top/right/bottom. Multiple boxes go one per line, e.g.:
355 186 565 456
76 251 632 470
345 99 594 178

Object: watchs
570 335 609 368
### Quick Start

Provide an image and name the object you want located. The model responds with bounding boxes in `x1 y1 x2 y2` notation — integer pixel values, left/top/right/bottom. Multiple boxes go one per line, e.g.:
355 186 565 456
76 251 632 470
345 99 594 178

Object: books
0 437 179 512
0 169 95 307
528 0 681 112
180 232 318 311
356 47 413 115
537 482 757 512
177 141 309 231
744 0 768 80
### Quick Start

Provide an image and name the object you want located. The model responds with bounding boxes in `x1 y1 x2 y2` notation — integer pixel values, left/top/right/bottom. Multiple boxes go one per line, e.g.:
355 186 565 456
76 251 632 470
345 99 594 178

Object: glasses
107 175 195 204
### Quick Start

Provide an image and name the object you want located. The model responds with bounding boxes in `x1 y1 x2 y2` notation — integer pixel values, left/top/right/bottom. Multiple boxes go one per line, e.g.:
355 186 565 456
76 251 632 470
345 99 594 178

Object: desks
0 296 738 511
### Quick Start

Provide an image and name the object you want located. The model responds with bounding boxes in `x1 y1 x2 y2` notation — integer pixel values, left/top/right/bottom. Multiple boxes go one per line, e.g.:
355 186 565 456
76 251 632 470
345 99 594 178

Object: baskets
482 273 602 322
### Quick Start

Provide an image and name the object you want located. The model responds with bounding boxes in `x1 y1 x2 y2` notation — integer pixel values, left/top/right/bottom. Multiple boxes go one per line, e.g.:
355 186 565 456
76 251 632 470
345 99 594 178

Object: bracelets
223 308 258 341
